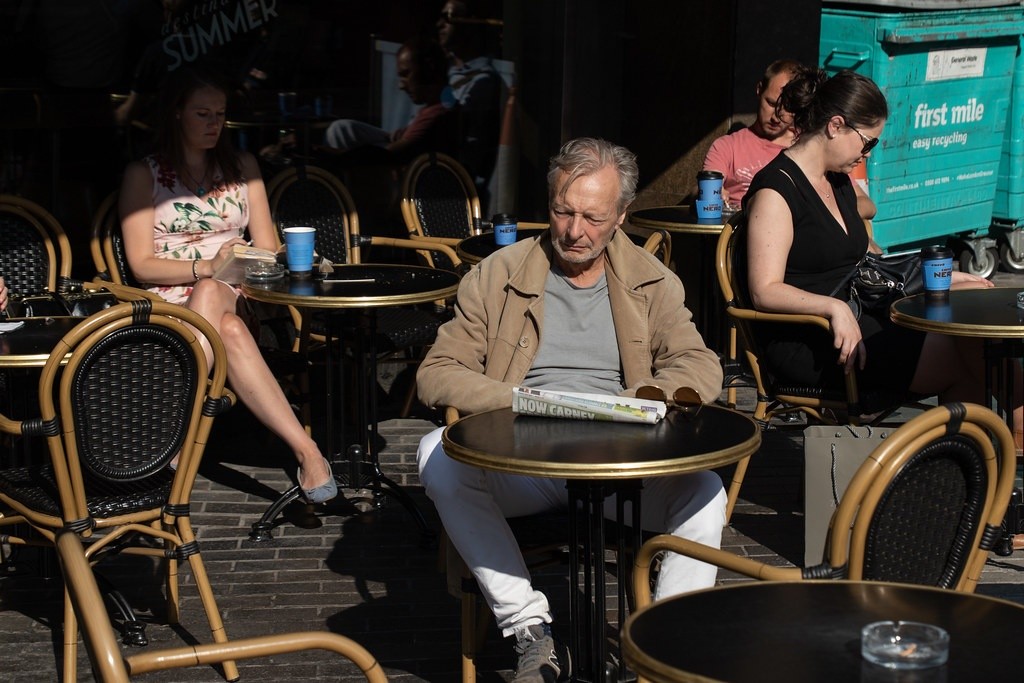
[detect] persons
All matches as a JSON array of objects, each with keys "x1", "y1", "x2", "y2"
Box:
[
  {"x1": 113, "y1": 0, "x2": 498, "y2": 187},
  {"x1": 742, "y1": 68, "x2": 1024, "y2": 455},
  {"x1": 119, "y1": 76, "x2": 338, "y2": 503},
  {"x1": 701, "y1": 58, "x2": 877, "y2": 219},
  {"x1": 417, "y1": 140, "x2": 727, "y2": 683},
  {"x1": 0, "y1": 277, "x2": 8, "y2": 311}
]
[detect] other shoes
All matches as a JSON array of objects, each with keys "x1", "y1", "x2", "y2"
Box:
[
  {"x1": 1010, "y1": 532, "x2": 1024, "y2": 550},
  {"x1": 297, "y1": 455, "x2": 337, "y2": 503},
  {"x1": 1012, "y1": 430, "x2": 1024, "y2": 456}
]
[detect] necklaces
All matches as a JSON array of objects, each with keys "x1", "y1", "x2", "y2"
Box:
[
  {"x1": 183, "y1": 160, "x2": 210, "y2": 197},
  {"x1": 787, "y1": 149, "x2": 829, "y2": 199}
]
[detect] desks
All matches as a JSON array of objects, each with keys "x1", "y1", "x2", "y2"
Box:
[
  {"x1": 628, "y1": 205, "x2": 731, "y2": 349},
  {"x1": 441, "y1": 398, "x2": 762, "y2": 683},
  {"x1": 889, "y1": 289, "x2": 1024, "y2": 556},
  {"x1": 621, "y1": 578, "x2": 1024, "y2": 683},
  {"x1": 0, "y1": 317, "x2": 147, "y2": 646},
  {"x1": 239, "y1": 263, "x2": 464, "y2": 549},
  {"x1": 456, "y1": 229, "x2": 547, "y2": 267}
]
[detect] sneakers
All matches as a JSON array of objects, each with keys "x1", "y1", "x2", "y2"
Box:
[{"x1": 513, "y1": 622, "x2": 561, "y2": 683}]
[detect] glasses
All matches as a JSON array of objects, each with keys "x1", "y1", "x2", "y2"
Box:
[{"x1": 844, "y1": 123, "x2": 879, "y2": 154}]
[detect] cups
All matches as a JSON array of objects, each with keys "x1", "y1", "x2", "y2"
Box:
[
  {"x1": 493, "y1": 212, "x2": 516, "y2": 246},
  {"x1": 283, "y1": 226, "x2": 316, "y2": 272},
  {"x1": 697, "y1": 170, "x2": 724, "y2": 201},
  {"x1": 919, "y1": 246, "x2": 953, "y2": 301}
]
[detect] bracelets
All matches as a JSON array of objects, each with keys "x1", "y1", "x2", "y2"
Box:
[{"x1": 193, "y1": 260, "x2": 198, "y2": 280}]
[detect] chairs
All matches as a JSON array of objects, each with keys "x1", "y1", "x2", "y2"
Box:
[{"x1": 1, "y1": 151, "x2": 1024, "y2": 683}]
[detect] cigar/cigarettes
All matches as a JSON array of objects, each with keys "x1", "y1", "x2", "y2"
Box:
[
  {"x1": 724, "y1": 198, "x2": 731, "y2": 210},
  {"x1": 246, "y1": 238, "x2": 254, "y2": 246}
]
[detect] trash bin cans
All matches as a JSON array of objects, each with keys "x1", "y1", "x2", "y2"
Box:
[
  {"x1": 993, "y1": 34, "x2": 1024, "y2": 274},
  {"x1": 819, "y1": 0, "x2": 1024, "y2": 280}
]
